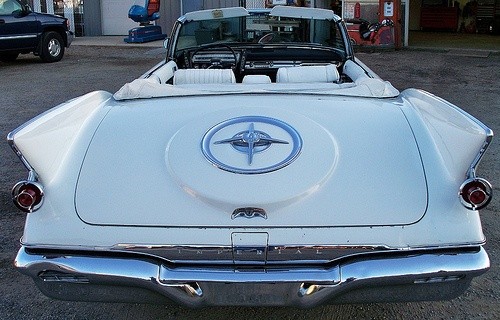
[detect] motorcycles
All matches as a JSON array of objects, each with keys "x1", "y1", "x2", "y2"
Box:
[{"x1": 336, "y1": 17, "x2": 394, "y2": 50}]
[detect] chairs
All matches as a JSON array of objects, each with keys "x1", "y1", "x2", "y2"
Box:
[
  {"x1": 173, "y1": 69, "x2": 235, "y2": 85},
  {"x1": 276, "y1": 65, "x2": 340, "y2": 84},
  {"x1": 242, "y1": 75, "x2": 271, "y2": 83}
]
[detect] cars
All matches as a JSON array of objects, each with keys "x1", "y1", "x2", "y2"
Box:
[
  {"x1": 6, "y1": 6, "x2": 495, "y2": 312},
  {"x1": 0, "y1": 0, "x2": 74, "y2": 64}
]
